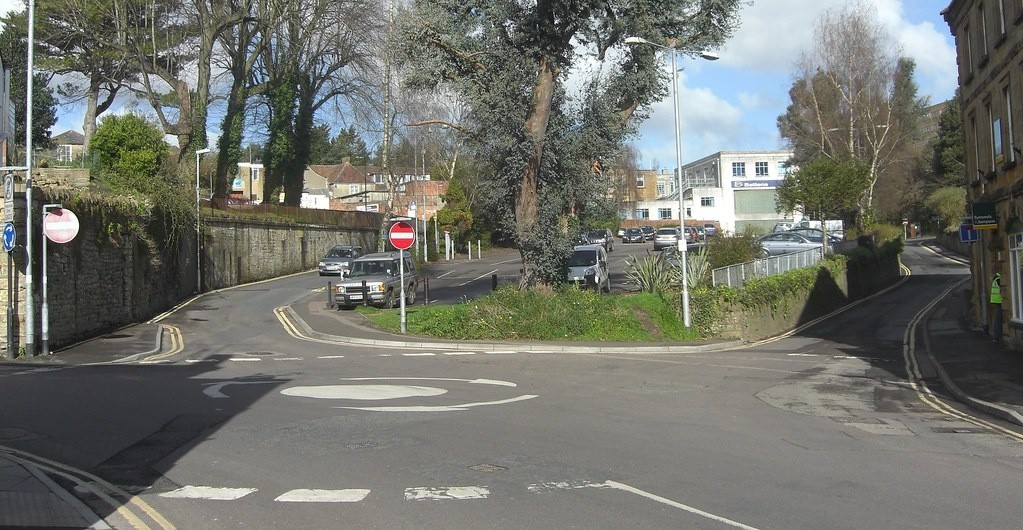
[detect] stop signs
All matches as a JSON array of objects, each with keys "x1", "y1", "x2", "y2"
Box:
[
  {"x1": 388, "y1": 221, "x2": 416, "y2": 251},
  {"x1": 42, "y1": 208, "x2": 79, "y2": 244},
  {"x1": 902, "y1": 220, "x2": 909, "y2": 226}
]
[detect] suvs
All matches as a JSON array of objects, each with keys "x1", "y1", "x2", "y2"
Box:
[
  {"x1": 317, "y1": 244, "x2": 364, "y2": 278},
  {"x1": 582, "y1": 226, "x2": 614, "y2": 254},
  {"x1": 653, "y1": 227, "x2": 681, "y2": 251},
  {"x1": 333, "y1": 250, "x2": 418, "y2": 310}
]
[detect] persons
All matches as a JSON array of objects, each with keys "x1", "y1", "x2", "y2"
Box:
[{"x1": 991, "y1": 273, "x2": 1003, "y2": 345}]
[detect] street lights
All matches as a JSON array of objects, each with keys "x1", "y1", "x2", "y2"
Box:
[
  {"x1": 829, "y1": 125, "x2": 889, "y2": 175},
  {"x1": 624, "y1": 36, "x2": 720, "y2": 328},
  {"x1": 196, "y1": 148, "x2": 211, "y2": 294}
]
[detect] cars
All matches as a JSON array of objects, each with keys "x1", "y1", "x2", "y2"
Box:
[
  {"x1": 639, "y1": 225, "x2": 657, "y2": 240},
  {"x1": 617, "y1": 227, "x2": 627, "y2": 238},
  {"x1": 752, "y1": 231, "x2": 824, "y2": 258},
  {"x1": 622, "y1": 227, "x2": 647, "y2": 244},
  {"x1": 771, "y1": 219, "x2": 847, "y2": 244},
  {"x1": 676, "y1": 225, "x2": 705, "y2": 244},
  {"x1": 702, "y1": 223, "x2": 718, "y2": 237}
]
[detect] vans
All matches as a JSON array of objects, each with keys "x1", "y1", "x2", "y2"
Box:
[{"x1": 564, "y1": 244, "x2": 610, "y2": 294}]
